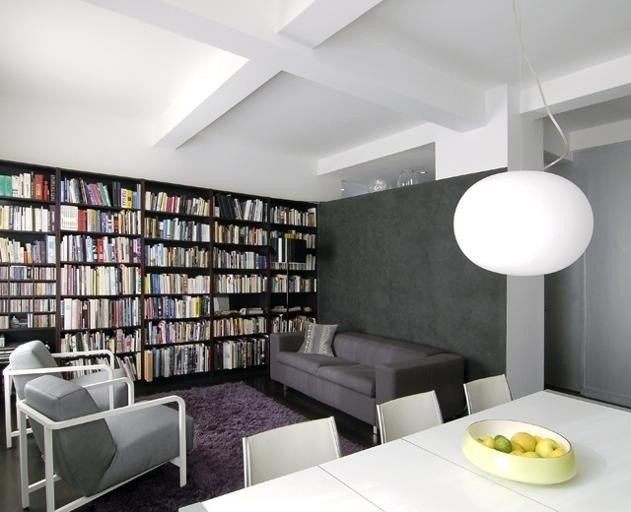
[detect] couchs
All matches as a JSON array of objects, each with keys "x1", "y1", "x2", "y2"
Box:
[{"x1": 270, "y1": 331, "x2": 465, "y2": 444}]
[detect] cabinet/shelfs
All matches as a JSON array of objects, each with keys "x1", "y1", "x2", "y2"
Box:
[{"x1": 0, "y1": 161, "x2": 320, "y2": 399}]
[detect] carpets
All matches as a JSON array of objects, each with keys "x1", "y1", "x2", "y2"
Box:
[{"x1": 92, "y1": 382, "x2": 368, "y2": 512}]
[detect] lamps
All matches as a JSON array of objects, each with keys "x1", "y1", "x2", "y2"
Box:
[{"x1": 453, "y1": 0, "x2": 593, "y2": 275}]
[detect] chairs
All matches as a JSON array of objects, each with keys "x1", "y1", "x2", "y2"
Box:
[
  {"x1": 2, "y1": 340, "x2": 128, "y2": 449},
  {"x1": 376, "y1": 390, "x2": 444, "y2": 444},
  {"x1": 464, "y1": 374, "x2": 513, "y2": 415},
  {"x1": 242, "y1": 416, "x2": 341, "y2": 487},
  {"x1": 16, "y1": 374, "x2": 194, "y2": 511}
]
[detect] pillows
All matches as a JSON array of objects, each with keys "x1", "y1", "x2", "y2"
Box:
[{"x1": 298, "y1": 321, "x2": 338, "y2": 358}]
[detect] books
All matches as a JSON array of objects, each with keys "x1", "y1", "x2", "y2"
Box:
[
  {"x1": 60, "y1": 176, "x2": 141, "y2": 381},
  {"x1": 1, "y1": 173, "x2": 56, "y2": 359},
  {"x1": 144, "y1": 190, "x2": 210, "y2": 381},
  {"x1": 212, "y1": 193, "x2": 317, "y2": 370}
]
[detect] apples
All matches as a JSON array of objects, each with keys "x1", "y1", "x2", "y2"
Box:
[
  {"x1": 508, "y1": 432, "x2": 566, "y2": 458},
  {"x1": 479, "y1": 437, "x2": 494, "y2": 448}
]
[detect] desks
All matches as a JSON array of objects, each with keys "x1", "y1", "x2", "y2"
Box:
[{"x1": 178, "y1": 389, "x2": 631, "y2": 512}]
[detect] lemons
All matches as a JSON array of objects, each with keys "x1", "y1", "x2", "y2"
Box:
[{"x1": 494, "y1": 435, "x2": 512, "y2": 453}]
